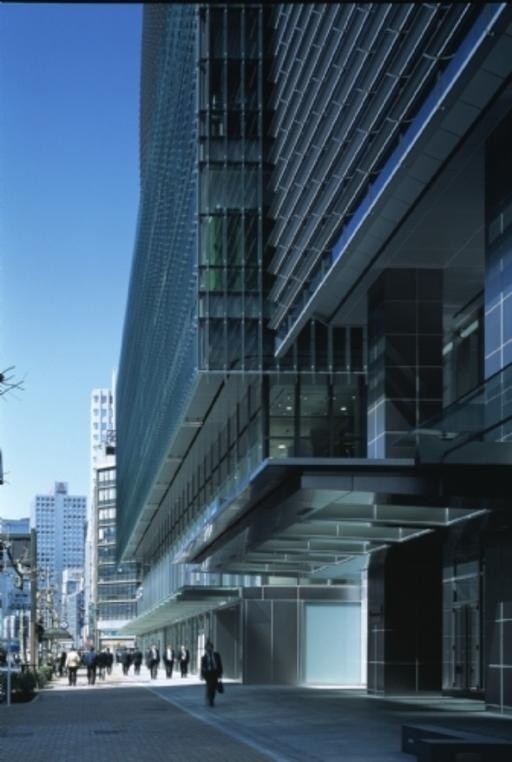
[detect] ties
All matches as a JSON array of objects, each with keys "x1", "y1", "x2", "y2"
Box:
[{"x1": 209, "y1": 655, "x2": 214, "y2": 670}]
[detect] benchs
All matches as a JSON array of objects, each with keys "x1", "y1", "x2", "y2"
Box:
[{"x1": 401, "y1": 721, "x2": 512, "y2": 762}]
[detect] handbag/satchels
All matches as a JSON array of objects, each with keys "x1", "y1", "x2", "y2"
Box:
[{"x1": 217, "y1": 682, "x2": 224, "y2": 694}]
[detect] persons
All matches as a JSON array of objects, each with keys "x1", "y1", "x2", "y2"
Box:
[
  {"x1": 57, "y1": 643, "x2": 191, "y2": 685},
  {"x1": 201, "y1": 641, "x2": 224, "y2": 708}
]
